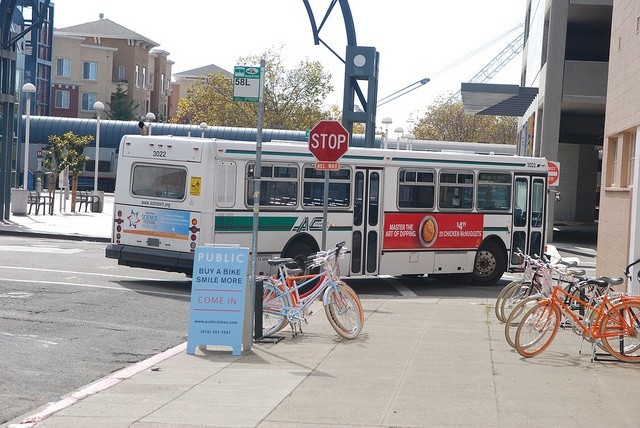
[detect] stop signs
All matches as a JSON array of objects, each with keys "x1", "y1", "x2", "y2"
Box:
[
  {"x1": 548, "y1": 160, "x2": 561, "y2": 189},
  {"x1": 308, "y1": 121, "x2": 349, "y2": 171}
]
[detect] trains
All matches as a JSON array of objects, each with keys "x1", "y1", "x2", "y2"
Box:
[{"x1": 11, "y1": 116, "x2": 603, "y2": 209}]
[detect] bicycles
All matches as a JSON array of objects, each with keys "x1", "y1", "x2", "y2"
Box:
[
  {"x1": 255, "y1": 241, "x2": 364, "y2": 340},
  {"x1": 495, "y1": 247, "x2": 640, "y2": 363}
]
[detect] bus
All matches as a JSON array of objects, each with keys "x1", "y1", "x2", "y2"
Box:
[{"x1": 106, "y1": 135, "x2": 548, "y2": 277}]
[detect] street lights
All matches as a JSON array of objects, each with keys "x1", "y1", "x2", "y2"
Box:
[
  {"x1": 22, "y1": 83, "x2": 36, "y2": 190},
  {"x1": 200, "y1": 122, "x2": 208, "y2": 138},
  {"x1": 145, "y1": 112, "x2": 156, "y2": 135},
  {"x1": 93, "y1": 101, "x2": 105, "y2": 191},
  {"x1": 394, "y1": 127, "x2": 404, "y2": 150},
  {"x1": 404, "y1": 134, "x2": 415, "y2": 150},
  {"x1": 382, "y1": 117, "x2": 392, "y2": 149},
  {"x1": 376, "y1": 77, "x2": 430, "y2": 108}
]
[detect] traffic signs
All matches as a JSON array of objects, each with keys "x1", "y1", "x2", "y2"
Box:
[{"x1": 233, "y1": 66, "x2": 261, "y2": 102}]
